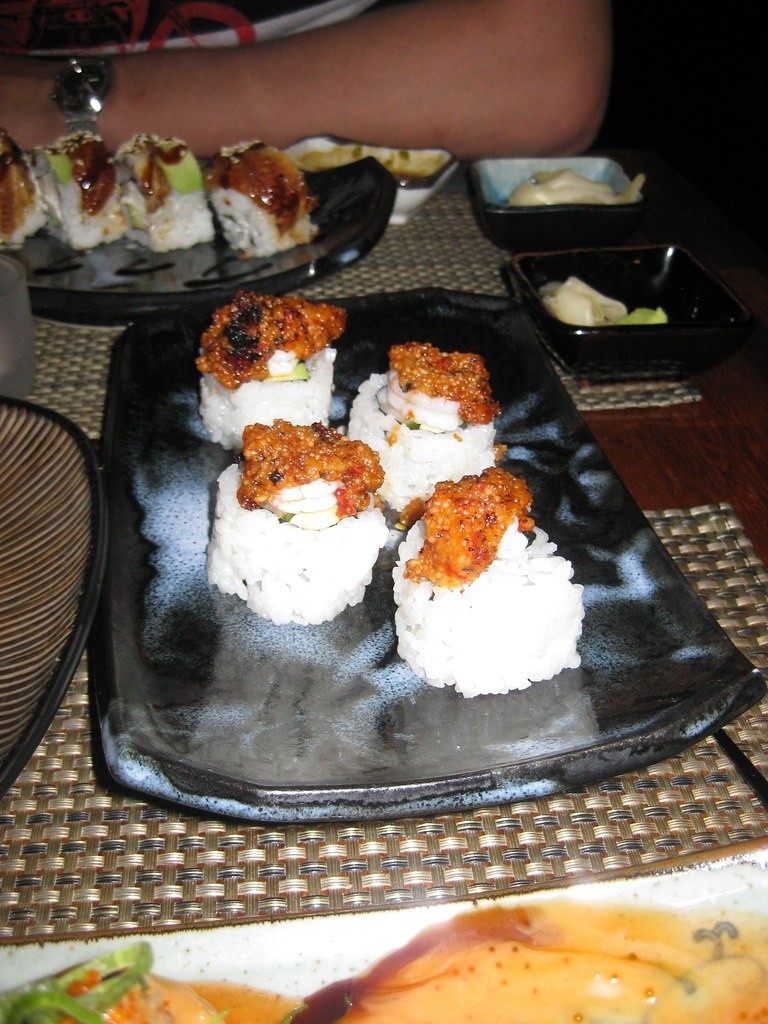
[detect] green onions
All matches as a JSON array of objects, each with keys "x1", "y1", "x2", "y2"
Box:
[{"x1": 0, "y1": 940, "x2": 153, "y2": 1024}]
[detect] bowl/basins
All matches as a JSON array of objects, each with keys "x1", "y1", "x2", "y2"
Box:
[
  {"x1": 275, "y1": 132, "x2": 458, "y2": 225},
  {"x1": 468, "y1": 154, "x2": 651, "y2": 252}
]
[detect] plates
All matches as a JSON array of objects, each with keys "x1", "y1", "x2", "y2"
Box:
[
  {"x1": 508, "y1": 243, "x2": 756, "y2": 386},
  {"x1": 1, "y1": 154, "x2": 397, "y2": 325},
  {"x1": 0, "y1": 823, "x2": 768, "y2": 1024},
  {"x1": 91, "y1": 283, "x2": 768, "y2": 820},
  {"x1": 0, "y1": 397, "x2": 103, "y2": 821}
]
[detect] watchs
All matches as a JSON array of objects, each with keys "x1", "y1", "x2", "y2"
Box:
[{"x1": 49, "y1": 54, "x2": 115, "y2": 135}]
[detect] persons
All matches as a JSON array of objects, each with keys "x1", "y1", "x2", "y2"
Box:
[{"x1": 0, "y1": 0, "x2": 613, "y2": 161}]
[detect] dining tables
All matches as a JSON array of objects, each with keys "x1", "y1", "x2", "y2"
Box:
[{"x1": 0, "y1": 149, "x2": 768, "y2": 947}]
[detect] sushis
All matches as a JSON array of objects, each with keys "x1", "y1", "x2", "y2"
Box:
[
  {"x1": 0, "y1": 127, "x2": 320, "y2": 259},
  {"x1": 194, "y1": 292, "x2": 583, "y2": 698}
]
